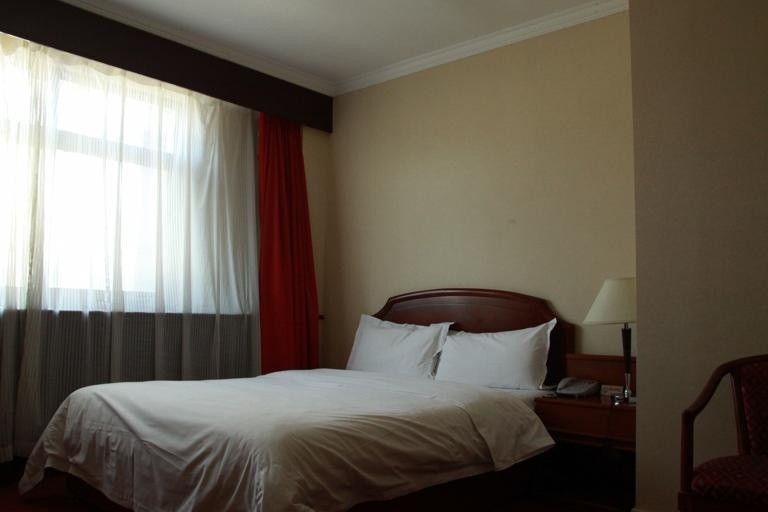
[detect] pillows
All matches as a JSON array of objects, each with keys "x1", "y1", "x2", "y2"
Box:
[
  {"x1": 435, "y1": 317, "x2": 557, "y2": 392},
  {"x1": 343, "y1": 313, "x2": 454, "y2": 380}
]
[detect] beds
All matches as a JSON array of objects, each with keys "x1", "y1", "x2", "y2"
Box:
[{"x1": 30, "y1": 288, "x2": 577, "y2": 510}]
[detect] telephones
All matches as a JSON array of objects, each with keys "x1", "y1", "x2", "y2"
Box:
[{"x1": 557, "y1": 378, "x2": 598, "y2": 396}]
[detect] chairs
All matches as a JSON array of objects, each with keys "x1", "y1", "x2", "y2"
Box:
[{"x1": 675, "y1": 349, "x2": 767, "y2": 511}]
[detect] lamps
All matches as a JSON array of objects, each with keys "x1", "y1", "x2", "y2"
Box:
[{"x1": 581, "y1": 271, "x2": 637, "y2": 404}]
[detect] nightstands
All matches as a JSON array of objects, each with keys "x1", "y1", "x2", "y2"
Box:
[{"x1": 535, "y1": 355, "x2": 637, "y2": 511}]
